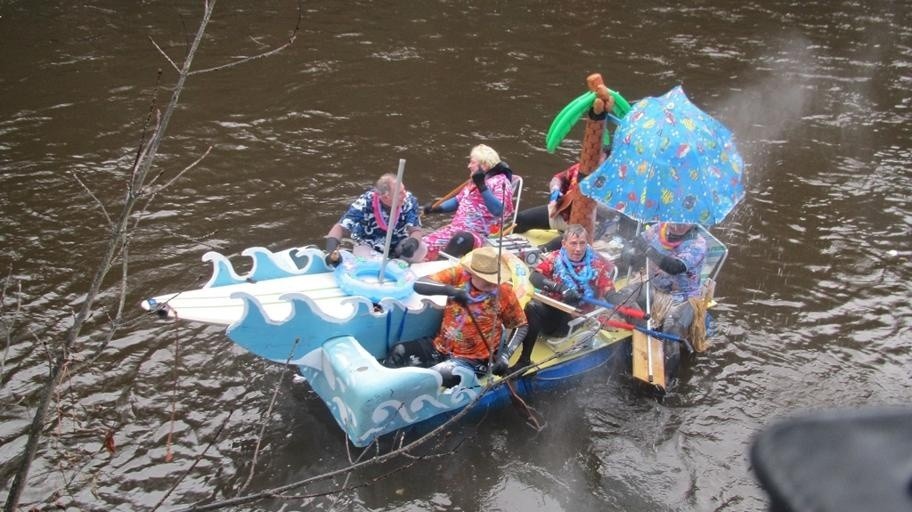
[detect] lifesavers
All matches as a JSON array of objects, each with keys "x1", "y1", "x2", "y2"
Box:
[
  {"x1": 334, "y1": 254, "x2": 417, "y2": 302},
  {"x1": 458, "y1": 246, "x2": 530, "y2": 310}
]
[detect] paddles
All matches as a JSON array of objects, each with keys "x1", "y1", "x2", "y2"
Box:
[{"x1": 632, "y1": 254, "x2": 665, "y2": 395}]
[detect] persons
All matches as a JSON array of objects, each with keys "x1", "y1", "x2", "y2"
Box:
[
  {"x1": 511, "y1": 149, "x2": 610, "y2": 233},
  {"x1": 624, "y1": 222, "x2": 708, "y2": 370},
  {"x1": 418, "y1": 143, "x2": 516, "y2": 261},
  {"x1": 511, "y1": 224, "x2": 628, "y2": 371},
  {"x1": 326, "y1": 172, "x2": 417, "y2": 267},
  {"x1": 387, "y1": 246, "x2": 532, "y2": 388}
]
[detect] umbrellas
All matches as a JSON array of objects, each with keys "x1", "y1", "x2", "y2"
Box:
[{"x1": 578, "y1": 85, "x2": 747, "y2": 238}]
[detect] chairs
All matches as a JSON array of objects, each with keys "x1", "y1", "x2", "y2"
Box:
[
  {"x1": 552, "y1": 248, "x2": 624, "y2": 358},
  {"x1": 638, "y1": 220, "x2": 728, "y2": 292},
  {"x1": 421, "y1": 173, "x2": 524, "y2": 263}
]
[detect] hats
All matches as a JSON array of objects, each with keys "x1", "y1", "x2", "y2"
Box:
[{"x1": 458, "y1": 246, "x2": 512, "y2": 287}]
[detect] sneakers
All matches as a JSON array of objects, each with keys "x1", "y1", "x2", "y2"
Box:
[{"x1": 500, "y1": 357, "x2": 534, "y2": 378}]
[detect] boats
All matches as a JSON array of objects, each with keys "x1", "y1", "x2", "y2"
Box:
[{"x1": 142, "y1": 200, "x2": 729, "y2": 449}]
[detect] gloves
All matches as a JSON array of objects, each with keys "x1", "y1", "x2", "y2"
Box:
[
  {"x1": 548, "y1": 186, "x2": 564, "y2": 203},
  {"x1": 423, "y1": 205, "x2": 444, "y2": 216},
  {"x1": 603, "y1": 285, "x2": 626, "y2": 306},
  {"x1": 401, "y1": 238, "x2": 421, "y2": 259},
  {"x1": 630, "y1": 233, "x2": 650, "y2": 254},
  {"x1": 550, "y1": 280, "x2": 582, "y2": 306},
  {"x1": 322, "y1": 235, "x2": 342, "y2": 254},
  {"x1": 471, "y1": 171, "x2": 489, "y2": 195},
  {"x1": 491, "y1": 345, "x2": 517, "y2": 376},
  {"x1": 446, "y1": 285, "x2": 471, "y2": 304}
]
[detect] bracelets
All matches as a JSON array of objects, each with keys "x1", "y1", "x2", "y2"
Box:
[
  {"x1": 557, "y1": 284, "x2": 563, "y2": 294},
  {"x1": 642, "y1": 245, "x2": 650, "y2": 253},
  {"x1": 551, "y1": 282, "x2": 558, "y2": 292},
  {"x1": 644, "y1": 246, "x2": 652, "y2": 256}
]
